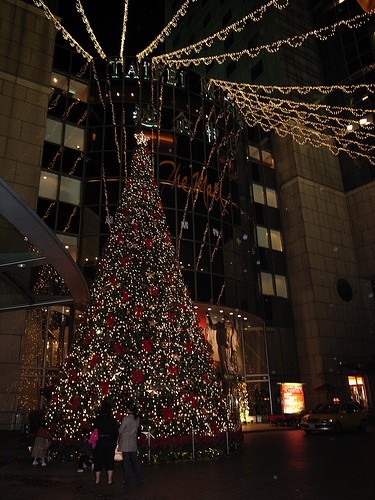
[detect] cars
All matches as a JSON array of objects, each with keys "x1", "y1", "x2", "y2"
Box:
[
  {"x1": 300, "y1": 400, "x2": 368, "y2": 435},
  {"x1": 268, "y1": 407, "x2": 311, "y2": 428}
]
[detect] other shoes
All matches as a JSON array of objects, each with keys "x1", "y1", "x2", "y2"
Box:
[
  {"x1": 32, "y1": 461, "x2": 37, "y2": 464},
  {"x1": 95, "y1": 480, "x2": 101, "y2": 485},
  {"x1": 137, "y1": 481, "x2": 145, "y2": 487},
  {"x1": 107, "y1": 480, "x2": 115, "y2": 486},
  {"x1": 41, "y1": 463, "x2": 47, "y2": 466},
  {"x1": 122, "y1": 480, "x2": 130, "y2": 487}
]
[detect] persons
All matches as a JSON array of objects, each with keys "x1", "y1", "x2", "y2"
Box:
[
  {"x1": 118, "y1": 404, "x2": 145, "y2": 487},
  {"x1": 32, "y1": 423, "x2": 51, "y2": 467},
  {"x1": 74, "y1": 408, "x2": 120, "y2": 485}
]
